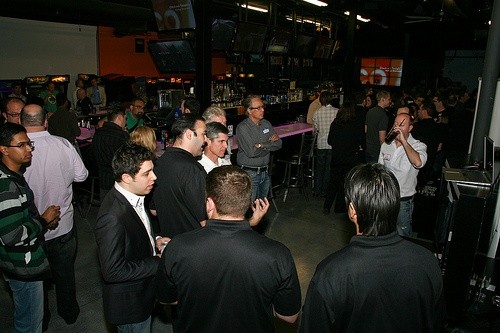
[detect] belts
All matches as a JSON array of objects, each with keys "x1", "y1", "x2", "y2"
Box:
[
  {"x1": 236, "y1": 163, "x2": 267, "y2": 172},
  {"x1": 399, "y1": 196, "x2": 413, "y2": 201}
]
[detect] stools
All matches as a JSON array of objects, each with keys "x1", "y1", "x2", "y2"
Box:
[{"x1": 273, "y1": 154, "x2": 310, "y2": 203}]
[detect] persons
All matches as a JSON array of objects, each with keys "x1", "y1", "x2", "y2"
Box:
[
  {"x1": 2, "y1": 97, "x2": 25, "y2": 125},
  {"x1": 19, "y1": 104, "x2": 89, "y2": 324},
  {"x1": 301, "y1": 163, "x2": 446, "y2": 333},
  {"x1": 94, "y1": 145, "x2": 171, "y2": 333},
  {"x1": 379, "y1": 112, "x2": 427, "y2": 237},
  {"x1": 10, "y1": 79, "x2": 107, "y2": 205},
  {"x1": 91, "y1": 95, "x2": 283, "y2": 239},
  {"x1": 306, "y1": 84, "x2": 476, "y2": 232},
  {"x1": 154, "y1": 166, "x2": 302, "y2": 333},
  {"x1": 0, "y1": 122, "x2": 61, "y2": 333}
]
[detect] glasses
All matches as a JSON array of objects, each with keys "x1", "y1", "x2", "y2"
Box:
[
  {"x1": 133, "y1": 104, "x2": 145, "y2": 111},
  {"x1": 6, "y1": 112, "x2": 20, "y2": 117},
  {"x1": 250, "y1": 105, "x2": 265, "y2": 110},
  {"x1": 3, "y1": 141, "x2": 35, "y2": 150}
]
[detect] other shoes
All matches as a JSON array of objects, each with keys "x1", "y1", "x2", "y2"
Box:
[
  {"x1": 334, "y1": 207, "x2": 348, "y2": 213},
  {"x1": 323, "y1": 208, "x2": 330, "y2": 213}
]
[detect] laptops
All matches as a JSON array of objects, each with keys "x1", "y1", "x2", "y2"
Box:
[{"x1": 443, "y1": 135, "x2": 495, "y2": 186}]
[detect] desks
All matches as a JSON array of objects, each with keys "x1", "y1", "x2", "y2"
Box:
[
  {"x1": 73, "y1": 109, "x2": 171, "y2": 158},
  {"x1": 228, "y1": 121, "x2": 313, "y2": 149}
]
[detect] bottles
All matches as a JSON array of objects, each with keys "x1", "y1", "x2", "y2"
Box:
[
  {"x1": 214, "y1": 83, "x2": 244, "y2": 108},
  {"x1": 87, "y1": 121, "x2": 90, "y2": 128},
  {"x1": 81, "y1": 120, "x2": 85, "y2": 127},
  {"x1": 262, "y1": 88, "x2": 303, "y2": 104},
  {"x1": 164, "y1": 134, "x2": 169, "y2": 150}
]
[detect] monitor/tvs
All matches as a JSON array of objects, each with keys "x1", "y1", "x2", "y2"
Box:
[{"x1": 147, "y1": 39, "x2": 198, "y2": 73}]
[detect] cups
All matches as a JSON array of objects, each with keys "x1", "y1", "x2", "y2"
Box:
[{"x1": 384, "y1": 125, "x2": 400, "y2": 145}]
